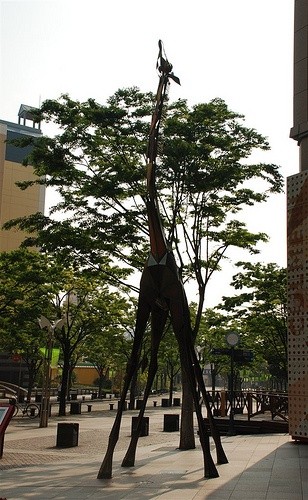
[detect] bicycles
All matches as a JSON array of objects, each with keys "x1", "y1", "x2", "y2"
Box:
[{"x1": 9, "y1": 393, "x2": 40, "y2": 419}]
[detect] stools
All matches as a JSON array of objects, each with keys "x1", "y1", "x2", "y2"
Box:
[
  {"x1": 164, "y1": 414, "x2": 180, "y2": 433},
  {"x1": 132, "y1": 416, "x2": 150, "y2": 437},
  {"x1": 161, "y1": 398, "x2": 169, "y2": 406},
  {"x1": 56, "y1": 423, "x2": 79, "y2": 448},
  {"x1": 118, "y1": 400, "x2": 128, "y2": 410},
  {"x1": 173, "y1": 398, "x2": 180, "y2": 406},
  {"x1": 136, "y1": 400, "x2": 145, "y2": 410}
]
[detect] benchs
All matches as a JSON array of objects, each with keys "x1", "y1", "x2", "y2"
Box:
[{"x1": 37, "y1": 403, "x2": 82, "y2": 416}]
